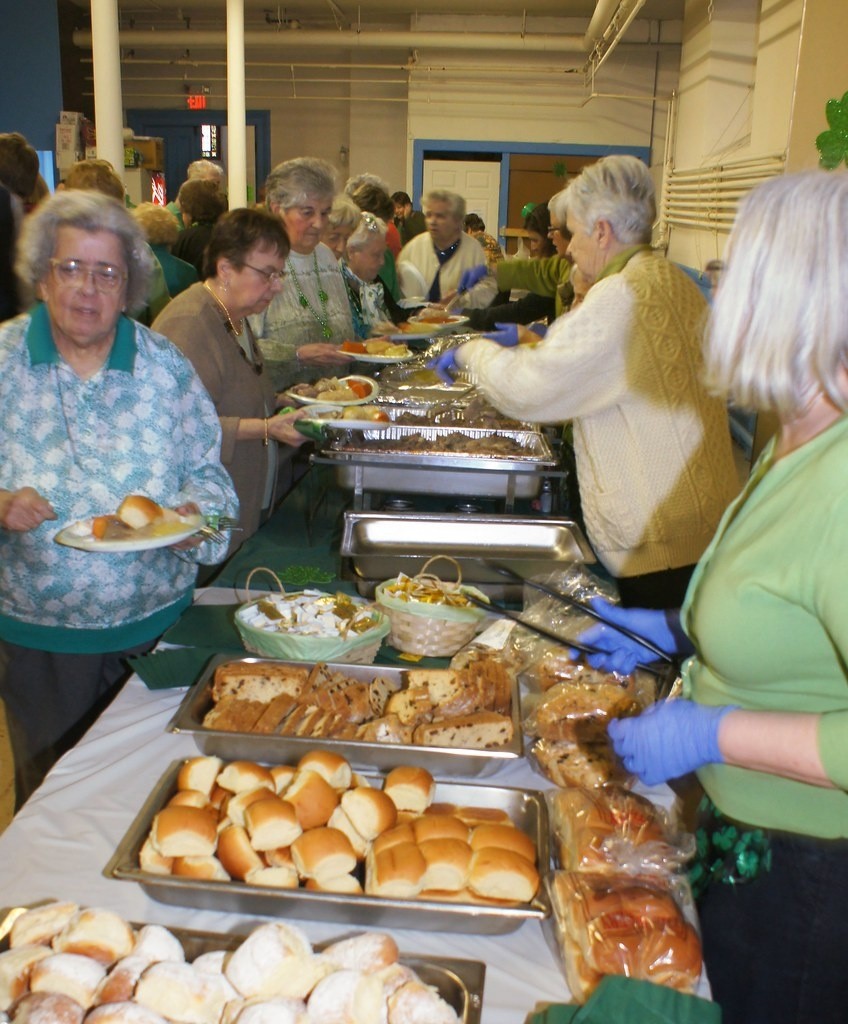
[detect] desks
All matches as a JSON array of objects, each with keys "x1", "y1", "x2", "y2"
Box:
[{"x1": 2, "y1": 463, "x2": 723, "y2": 1024}]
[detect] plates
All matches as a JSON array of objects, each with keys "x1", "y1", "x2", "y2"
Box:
[
  {"x1": 407, "y1": 315, "x2": 470, "y2": 329},
  {"x1": 367, "y1": 328, "x2": 443, "y2": 344},
  {"x1": 299, "y1": 419, "x2": 390, "y2": 429},
  {"x1": 336, "y1": 348, "x2": 414, "y2": 363},
  {"x1": 285, "y1": 375, "x2": 380, "y2": 405},
  {"x1": 54, "y1": 513, "x2": 207, "y2": 552}
]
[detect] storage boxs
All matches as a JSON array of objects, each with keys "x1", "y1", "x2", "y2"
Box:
[
  {"x1": 124, "y1": 137, "x2": 165, "y2": 172},
  {"x1": 54, "y1": 111, "x2": 98, "y2": 169}
]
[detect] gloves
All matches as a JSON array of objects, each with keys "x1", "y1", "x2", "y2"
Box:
[
  {"x1": 426, "y1": 343, "x2": 462, "y2": 386},
  {"x1": 455, "y1": 264, "x2": 489, "y2": 295},
  {"x1": 480, "y1": 322, "x2": 521, "y2": 348},
  {"x1": 606, "y1": 698, "x2": 741, "y2": 788},
  {"x1": 566, "y1": 595, "x2": 695, "y2": 674},
  {"x1": 529, "y1": 322, "x2": 549, "y2": 338}
]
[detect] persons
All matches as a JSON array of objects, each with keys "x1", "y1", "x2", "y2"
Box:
[
  {"x1": 396, "y1": 190, "x2": 488, "y2": 303},
  {"x1": 0, "y1": 133, "x2": 229, "y2": 343},
  {"x1": 426, "y1": 156, "x2": 742, "y2": 606},
  {"x1": 148, "y1": 207, "x2": 316, "y2": 587},
  {"x1": 568, "y1": 171, "x2": 848, "y2": 1024},
  {"x1": 0, "y1": 190, "x2": 239, "y2": 817},
  {"x1": 251, "y1": 152, "x2": 427, "y2": 393}
]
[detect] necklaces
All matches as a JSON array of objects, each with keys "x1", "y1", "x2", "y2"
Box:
[
  {"x1": 205, "y1": 281, "x2": 244, "y2": 335},
  {"x1": 285, "y1": 250, "x2": 334, "y2": 343}
]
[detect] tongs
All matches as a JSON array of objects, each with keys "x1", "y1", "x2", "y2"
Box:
[{"x1": 465, "y1": 559, "x2": 679, "y2": 677}]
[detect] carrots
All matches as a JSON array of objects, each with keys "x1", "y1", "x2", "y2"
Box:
[
  {"x1": 372, "y1": 410, "x2": 389, "y2": 423},
  {"x1": 346, "y1": 379, "x2": 373, "y2": 399},
  {"x1": 340, "y1": 341, "x2": 369, "y2": 353}
]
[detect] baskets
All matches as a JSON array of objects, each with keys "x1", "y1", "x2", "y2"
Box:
[
  {"x1": 234, "y1": 567, "x2": 391, "y2": 666},
  {"x1": 374, "y1": 554, "x2": 489, "y2": 658}
]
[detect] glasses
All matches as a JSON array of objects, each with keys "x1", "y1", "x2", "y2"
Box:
[
  {"x1": 547, "y1": 227, "x2": 565, "y2": 234},
  {"x1": 46, "y1": 260, "x2": 132, "y2": 295},
  {"x1": 244, "y1": 263, "x2": 292, "y2": 287}
]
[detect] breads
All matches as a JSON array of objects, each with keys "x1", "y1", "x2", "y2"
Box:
[
  {"x1": 116, "y1": 495, "x2": 163, "y2": 529},
  {"x1": 0, "y1": 902, "x2": 462, "y2": 1024},
  {"x1": 525, "y1": 650, "x2": 705, "y2": 995},
  {"x1": 137, "y1": 750, "x2": 538, "y2": 909},
  {"x1": 203, "y1": 641, "x2": 513, "y2": 749}
]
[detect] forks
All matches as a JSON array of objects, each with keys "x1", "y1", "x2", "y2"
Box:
[
  {"x1": 205, "y1": 516, "x2": 240, "y2": 532},
  {"x1": 198, "y1": 524, "x2": 229, "y2": 545}
]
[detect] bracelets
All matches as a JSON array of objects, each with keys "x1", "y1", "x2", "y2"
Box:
[{"x1": 263, "y1": 418, "x2": 268, "y2": 449}]
